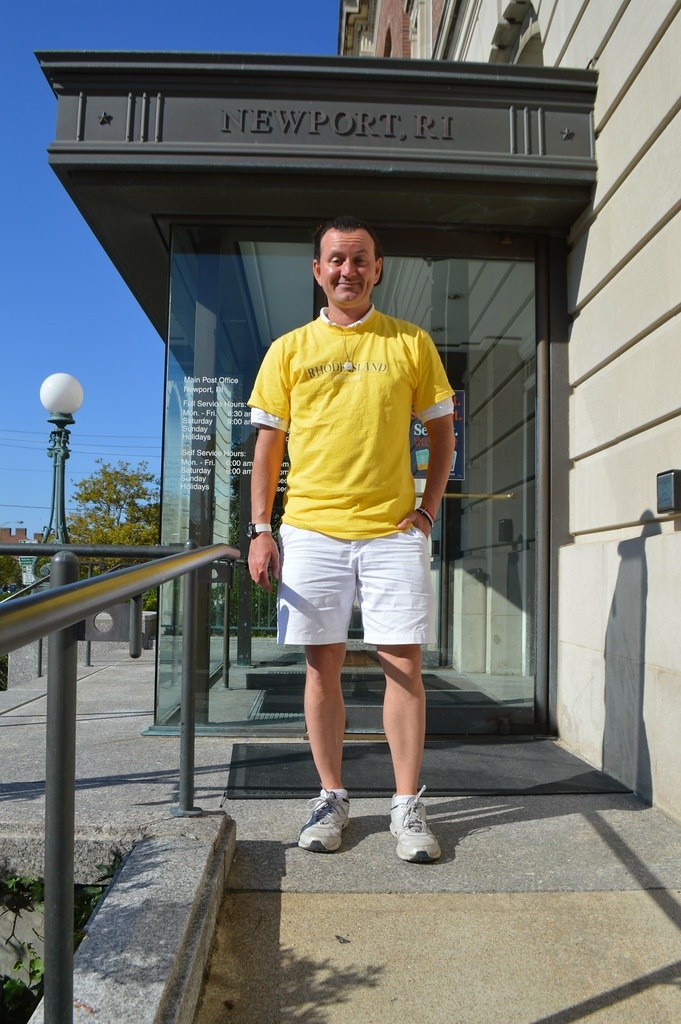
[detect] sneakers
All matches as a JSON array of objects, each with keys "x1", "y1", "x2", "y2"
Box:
[
  {"x1": 298, "y1": 783, "x2": 350, "y2": 853},
  {"x1": 389, "y1": 785, "x2": 442, "y2": 862}
]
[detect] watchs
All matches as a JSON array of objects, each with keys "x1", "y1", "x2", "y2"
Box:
[{"x1": 246, "y1": 522, "x2": 271, "y2": 538}]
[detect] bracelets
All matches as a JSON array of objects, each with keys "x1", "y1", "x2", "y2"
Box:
[{"x1": 417, "y1": 507, "x2": 434, "y2": 526}]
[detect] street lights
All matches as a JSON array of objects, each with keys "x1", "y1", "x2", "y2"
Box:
[{"x1": 33, "y1": 373, "x2": 83, "y2": 582}]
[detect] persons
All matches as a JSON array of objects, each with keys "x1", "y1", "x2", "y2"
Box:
[{"x1": 248, "y1": 215, "x2": 456, "y2": 860}]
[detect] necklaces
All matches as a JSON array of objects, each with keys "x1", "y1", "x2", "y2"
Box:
[{"x1": 340, "y1": 326, "x2": 355, "y2": 370}]
